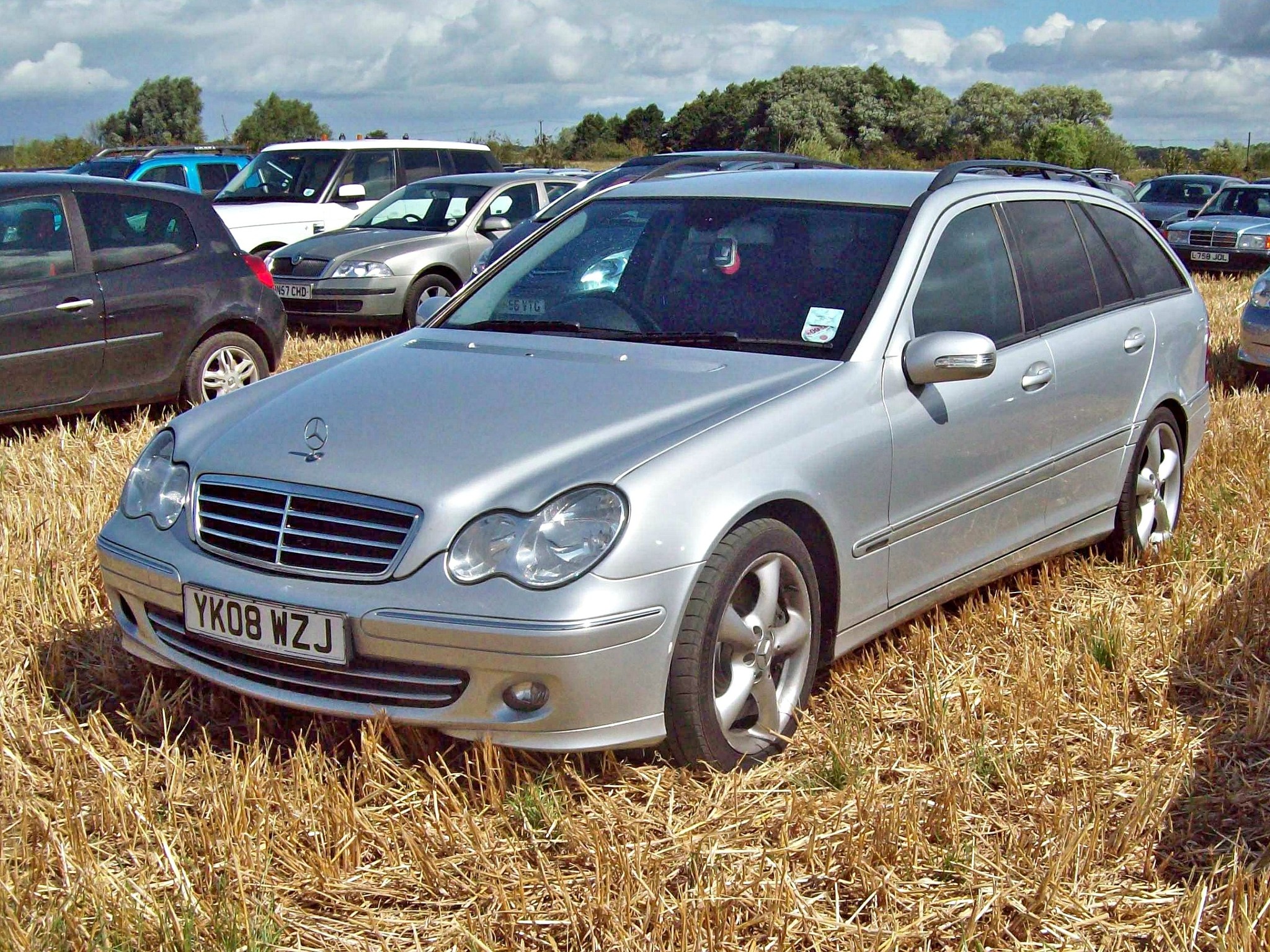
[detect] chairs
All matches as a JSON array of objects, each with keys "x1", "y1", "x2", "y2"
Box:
[
  {"x1": 18, "y1": 208, "x2": 55, "y2": 249},
  {"x1": 465, "y1": 196, "x2": 490, "y2": 217},
  {"x1": 1187, "y1": 187, "x2": 1204, "y2": 197},
  {"x1": 1239, "y1": 194, "x2": 1259, "y2": 214}
]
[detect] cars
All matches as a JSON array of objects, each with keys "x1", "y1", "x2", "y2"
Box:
[
  {"x1": 465, "y1": 149, "x2": 864, "y2": 323},
  {"x1": 503, "y1": 162, "x2": 609, "y2": 201},
  {"x1": 1133, "y1": 175, "x2": 1270, "y2": 240},
  {"x1": 259, "y1": 172, "x2": 592, "y2": 333},
  {"x1": 0, "y1": 171, "x2": 288, "y2": 422},
  {"x1": 1164, "y1": 184, "x2": 1270, "y2": 268},
  {"x1": 1236, "y1": 266, "x2": 1270, "y2": 371}
]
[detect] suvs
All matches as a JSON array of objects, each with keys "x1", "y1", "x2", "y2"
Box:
[
  {"x1": 0, "y1": 145, "x2": 267, "y2": 247},
  {"x1": 97, "y1": 157, "x2": 1209, "y2": 782},
  {"x1": 1021, "y1": 166, "x2": 1146, "y2": 233}
]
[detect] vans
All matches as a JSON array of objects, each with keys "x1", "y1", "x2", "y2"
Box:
[{"x1": 212, "y1": 138, "x2": 514, "y2": 265}]
